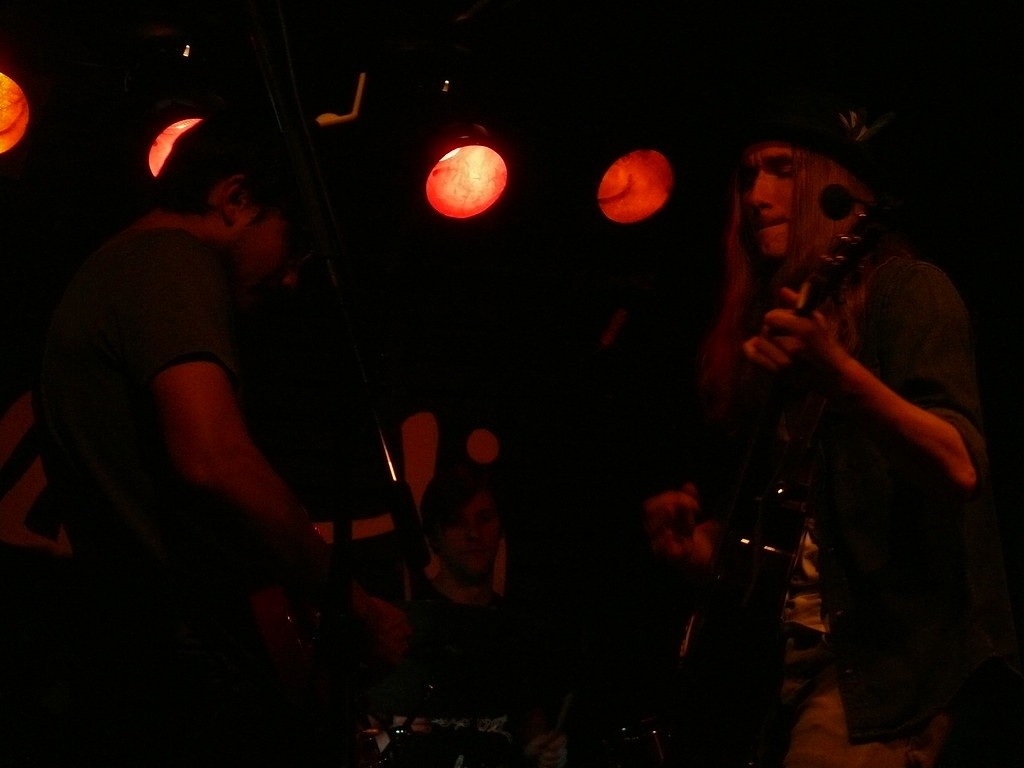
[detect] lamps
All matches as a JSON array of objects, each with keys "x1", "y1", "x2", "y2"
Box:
[{"x1": 417, "y1": 123, "x2": 509, "y2": 223}]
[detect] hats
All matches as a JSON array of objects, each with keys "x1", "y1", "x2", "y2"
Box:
[{"x1": 758, "y1": 88, "x2": 887, "y2": 196}]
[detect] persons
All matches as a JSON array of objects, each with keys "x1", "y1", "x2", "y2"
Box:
[
  {"x1": 641, "y1": 95, "x2": 1017, "y2": 768},
  {"x1": 356, "y1": 465, "x2": 567, "y2": 768},
  {"x1": 41, "y1": 93, "x2": 414, "y2": 768}
]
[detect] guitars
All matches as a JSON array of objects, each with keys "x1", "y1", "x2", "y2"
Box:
[{"x1": 684, "y1": 194, "x2": 902, "y2": 684}]
